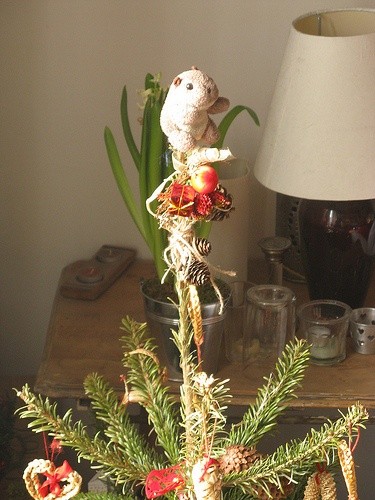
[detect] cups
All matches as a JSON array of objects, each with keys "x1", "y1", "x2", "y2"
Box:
[
  {"x1": 296, "y1": 299, "x2": 352, "y2": 366},
  {"x1": 224, "y1": 281, "x2": 256, "y2": 365},
  {"x1": 242, "y1": 284, "x2": 297, "y2": 382}
]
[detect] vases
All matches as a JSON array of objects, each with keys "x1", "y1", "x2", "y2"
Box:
[{"x1": 139, "y1": 274, "x2": 232, "y2": 383}]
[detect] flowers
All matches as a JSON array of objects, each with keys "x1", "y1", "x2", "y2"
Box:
[{"x1": 105, "y1": 72, "x2": 261, "y2": 294}]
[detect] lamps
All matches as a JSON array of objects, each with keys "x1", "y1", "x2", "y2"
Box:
[{"x1": 252, "y1": 7, "x2": 375, "y2": 319}]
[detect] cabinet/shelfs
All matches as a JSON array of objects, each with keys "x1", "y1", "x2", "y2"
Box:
[{"x1": 33, "y1": 257, "x2": 375, "y2": 500}]
